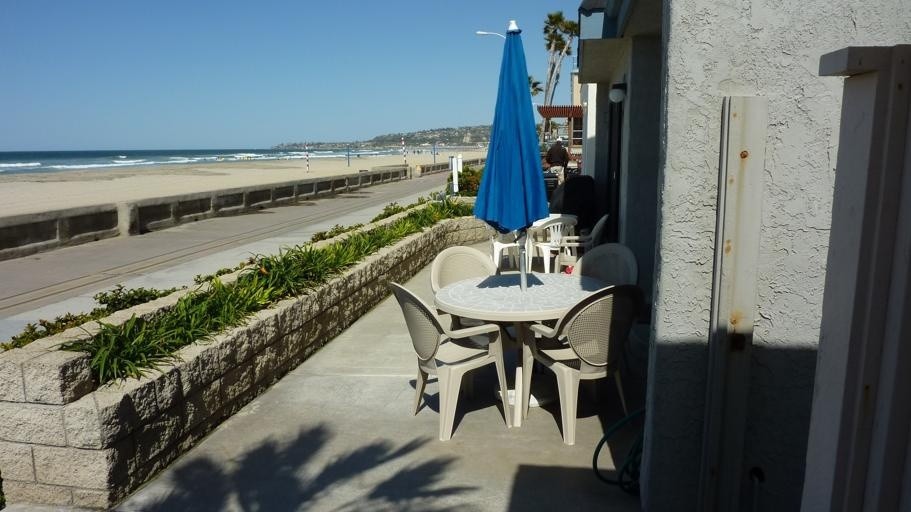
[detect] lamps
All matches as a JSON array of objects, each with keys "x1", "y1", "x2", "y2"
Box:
[{"x1": 608, "y1": 82, "x2": 627, "y2": 103}]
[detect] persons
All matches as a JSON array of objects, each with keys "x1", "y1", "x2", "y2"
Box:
[
  {"x1": 544, "y1": 137, "x2": 568, "y2": 189},
  {"x1": 407, "y1": 150, "x2": 440, "y2": 155}
]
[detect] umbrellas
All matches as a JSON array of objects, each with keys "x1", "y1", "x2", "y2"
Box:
[{"x1": 470, "y1": 18, "x2": 550, "y2": 294}]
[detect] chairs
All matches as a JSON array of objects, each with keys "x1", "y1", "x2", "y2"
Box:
[
  {"x1": 388, "y1": 281, "x2": 512, "y2": 441},
  {"x1": 571, "y1": 243, "x2": 639, "y2": 287},
  {"x1": 522, "y1": 283, "x2": 643, "y2": 447},
  {"x1": 488, "y1": 202, "x2": 609, "y2": 274},
  {"x1": 431, "y1": 246, "x2": 500, "y2": 329}
]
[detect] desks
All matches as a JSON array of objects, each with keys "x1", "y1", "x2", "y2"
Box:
[{"x1": 434, "y1": 272, "x2": 609, "y2": 427}]
